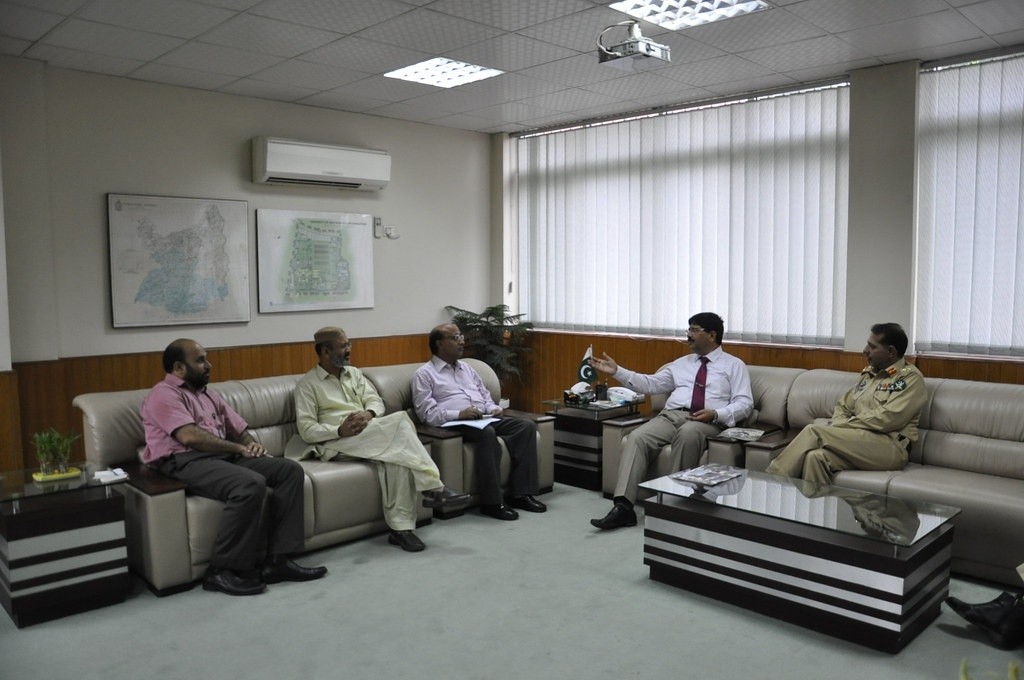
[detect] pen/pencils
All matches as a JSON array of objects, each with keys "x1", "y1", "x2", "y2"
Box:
[
  {"x1": 482, "y1": 415, "x2": 493, "y2": 418},
  {"x1": 107, "y1": 467, "x2": 119, "y2": 476},
  {"x1": 352, "y1": 387, "x2": 357, "y2": 396}
]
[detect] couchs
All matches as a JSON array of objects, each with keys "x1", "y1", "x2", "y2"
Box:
[
  {"x1": 602, "y1": 364, "x2": 1024, "y2": 588},
  {"x1": 73, "y1": 359, "x2": 556, "y2": 597}
]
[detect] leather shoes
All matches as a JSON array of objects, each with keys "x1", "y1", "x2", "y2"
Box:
[
  {"x1": 942, "y1": 588, "x2": 1024, "y2": 651},
  {"x1": 203, "y1": 570, "x2": 265, "y2": 596},
  {"x1": 421, "y1": 486, "x2": 472, "y2": 507},
  {"x1": 389, "y1": 529, "x2": 425, "y2": 553},
  {"x1": 590, "y1": 504, "x2": 637, "y2": 529},
  {"x1": 263, "y1": 558, "x2": 329, "y2": 585},
  {"x1": 475, "y1": 503, "x2": 519, "y2": 521},
  {"x1": 503, "y1": 492, "x2": 547, "y2": 512}
]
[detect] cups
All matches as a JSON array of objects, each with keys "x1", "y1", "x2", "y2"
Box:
[{"x1": 596, "y1": 385, "x2": 607, "y2": 399}]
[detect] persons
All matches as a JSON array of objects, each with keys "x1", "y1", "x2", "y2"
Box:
[
  {"x1": 284, "y1": 327, "x2": 473, "y2": 552},
  {"x1": 587, "y1": 313, "x2": 754, "y2": 529},
  {"x1": 412, "y1": 323, "x2": 546, "y2": 520},
  {"x1": 138, "y1": 339, "x2": 327, "y2": 596},
  {"x1": 764, "y1": 323, "x2": 927, "y2": 485},
  {"x1": 945, "y1": 562, "x2": 1024, "y2": 648}
]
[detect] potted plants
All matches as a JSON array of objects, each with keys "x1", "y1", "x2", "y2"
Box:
[{"x1": 33, "y1": 430, "x2": 79, "y2": 474}]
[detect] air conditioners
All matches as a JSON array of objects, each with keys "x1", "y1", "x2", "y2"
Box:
[{"x1": 251, "y1": 136, "x2": 391, "y2": 192}]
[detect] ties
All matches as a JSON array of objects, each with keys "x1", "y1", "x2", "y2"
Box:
[{"x1": 690, "y1": 356, "x2": 711, "y2": 418}]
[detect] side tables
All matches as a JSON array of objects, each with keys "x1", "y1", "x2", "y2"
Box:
[
  {"x1": 542, "y1": 393, "x2": 644, "y2": 492},
  {"x1": 1, "y1": 467, "x2": 128, "y2": 630}
]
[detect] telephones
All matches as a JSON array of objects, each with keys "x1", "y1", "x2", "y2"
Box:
[{"x1": 607, "y1": 386, "x2": 645, "y2": 404}]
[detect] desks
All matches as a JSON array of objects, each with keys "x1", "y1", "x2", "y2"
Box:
[{"x1": 637, "y1": 463, "x2": 961, "y2": 655}]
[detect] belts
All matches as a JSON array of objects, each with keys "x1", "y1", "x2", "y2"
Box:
[{"x1": 672, "y1": 407, "x2": 690, "y2": 413}]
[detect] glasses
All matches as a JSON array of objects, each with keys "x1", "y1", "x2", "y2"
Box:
[
  {"x1": 441, "y1": 335, "x2": 464, "y2": 341},
  {"x1": 332, "y1": 339, "x2": 352, "y2": 349}
]
